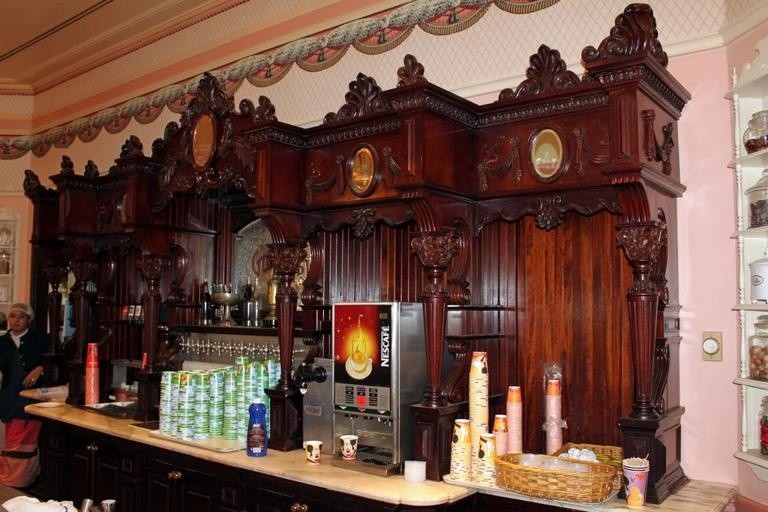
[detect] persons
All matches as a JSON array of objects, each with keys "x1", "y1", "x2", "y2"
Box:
[{"x1": 0, "y1": 302, "x2": 52, "y2": 488}]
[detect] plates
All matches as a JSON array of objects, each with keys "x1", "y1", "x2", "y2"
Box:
[{"x1": 345, "y1": 356, "x2": 373, "y2": 380}]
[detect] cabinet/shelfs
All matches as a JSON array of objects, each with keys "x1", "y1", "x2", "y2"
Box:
[
  {"x1": 0, "y1": 208, "x2": 21, "y2": 336},
  {"x1": 724, "y1": 36, "x2": 768, "y2": 512}
]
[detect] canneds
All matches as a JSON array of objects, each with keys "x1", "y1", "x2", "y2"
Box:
[{"x1": 159, "y1": 357, "x2": 281, "y2": 440}]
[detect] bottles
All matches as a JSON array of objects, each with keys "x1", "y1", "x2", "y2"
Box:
[
  {"x1": 745, "y1": 168, "x2": 767, "y2": 229},
  {"x1": 748, "y1": 248, "x2": 767, "y2": 306},
  {"x1": 743, "y1": 109, "x2": 768, "y2": 154},
  {"x1": 200, "y1": 275, "x2": 264, "y2": 326},
  {"x1": 747, "y1": 315, "x2": 768, "y2": 382},
  {"x1": 246, "y1": 398, "x2": 267, "y2": 457}
]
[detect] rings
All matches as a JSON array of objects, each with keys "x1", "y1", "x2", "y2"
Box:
[{"x1": 31, "y1": 378, "x2": 35, "y2": 382}]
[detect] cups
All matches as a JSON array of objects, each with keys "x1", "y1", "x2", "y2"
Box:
[
  {"x1": 157, "y1": 355, "x2": 282, "y2": 442},
  {"x1": 303, "y1": 439, "x2": 323, "y2": 465},
  {"x1": 449, "y1": 351, "x2": 523, "y2": 487},
  {"x1": 339, "y1": 434, "x2": 358, "y2": 461},
  {"x1": 622, "y1": 456, "x2": 650, "y2": 509},
  {"x1": 84, "y1": 343, "x2": 100, "y2": 407},
  {"x1": 545, "y1": 379, "x2": 563, "y2": 456}
]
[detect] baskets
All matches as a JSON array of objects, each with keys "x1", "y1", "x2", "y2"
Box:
[
  {"x1": 553, "y1": 441, "x2": 626, "y2": 491},
  {"x1": 494, "y1": 453, "x2": 617, "y2": 502}
]
